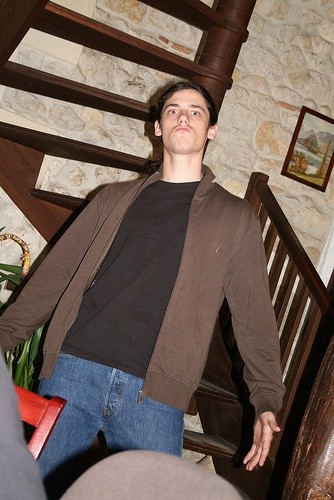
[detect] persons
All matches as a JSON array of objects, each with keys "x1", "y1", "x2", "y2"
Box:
[{"x1": 0, "y1": 80, "x2": 287, "y2": 500}]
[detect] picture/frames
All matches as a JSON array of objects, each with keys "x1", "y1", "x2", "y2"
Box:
[{"x1": 280, "y1": 105, "x2": 334, "y2": 192}]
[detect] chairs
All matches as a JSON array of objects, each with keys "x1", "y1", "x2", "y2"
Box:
[{"x1": 13, "y1": 383, "x2": 69, "y2": 461}]
